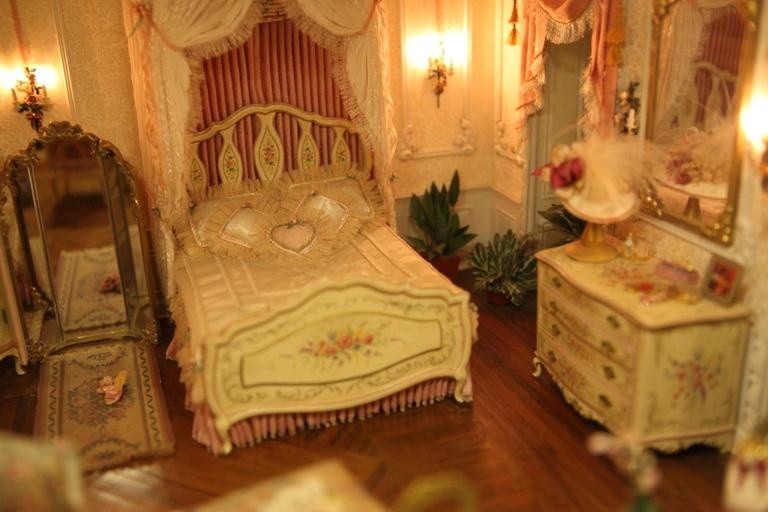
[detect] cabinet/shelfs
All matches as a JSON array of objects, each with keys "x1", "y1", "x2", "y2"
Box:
[{"x1": 532, "y1": 241, "x2": 752, "y2": 478}]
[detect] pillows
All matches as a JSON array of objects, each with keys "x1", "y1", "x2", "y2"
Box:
[{"x1": 175, "y1": 157, "x2": 392, "y2": 272}]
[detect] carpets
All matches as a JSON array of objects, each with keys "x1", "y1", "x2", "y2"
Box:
[
  {"x1": 31, "y1": 343, "x2": 175, "y2": 474},
  {"x1": 56, "y1": 245, "x2": 127, "y2": 333}
]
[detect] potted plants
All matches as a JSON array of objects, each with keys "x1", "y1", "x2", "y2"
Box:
[{"x1": 406, "y1": 167, "x2": 538, "y2": 309}]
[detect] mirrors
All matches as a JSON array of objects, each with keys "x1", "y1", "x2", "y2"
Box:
[
  {"x1": 637, "y1": 1, "x2": 764, "y2": 246},
  {"x1": 0, "y1": 120, "x2": 166, "y2": 366}
]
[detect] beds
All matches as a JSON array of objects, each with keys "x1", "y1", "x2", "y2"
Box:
[{"x1": 159, "y1": 103, "x2": 479, "y2": 457}]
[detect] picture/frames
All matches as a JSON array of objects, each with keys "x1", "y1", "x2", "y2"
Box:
[{"x1": 701, "y1": 253, "x2": 744, "y2": 307}]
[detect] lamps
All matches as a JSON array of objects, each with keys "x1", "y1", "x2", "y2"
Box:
[{"x1": 1, "y1": 65, "x2": 60, "y2": 135}]
